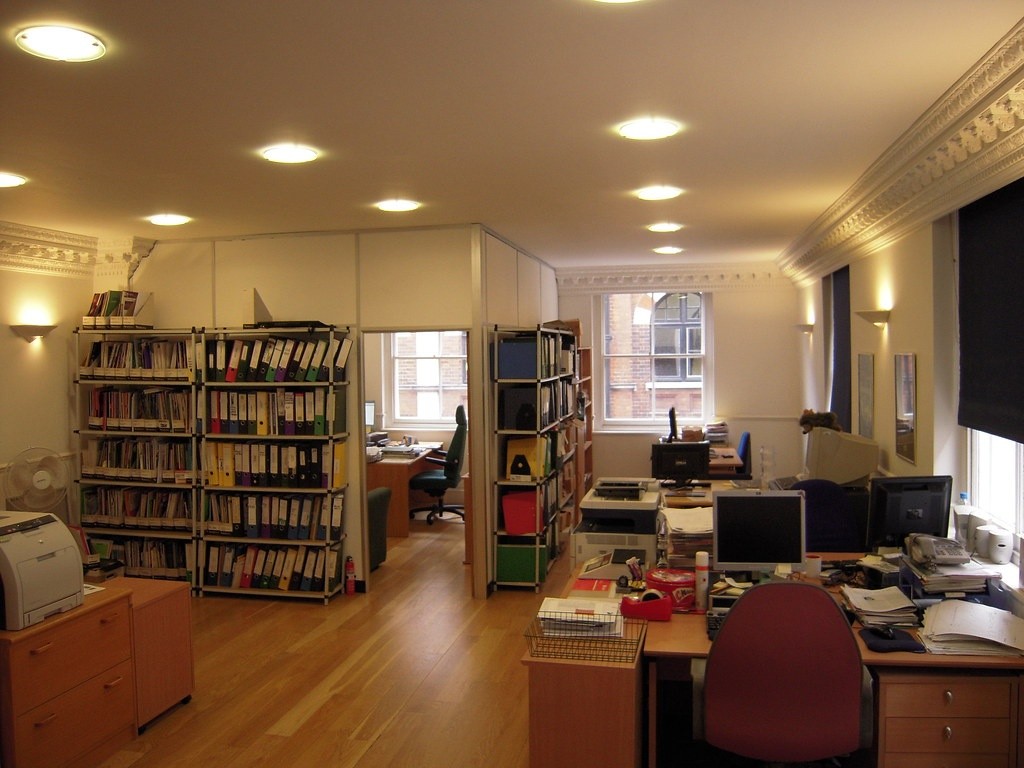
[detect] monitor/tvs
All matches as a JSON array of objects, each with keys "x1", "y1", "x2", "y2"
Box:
[
  {"x1": 666, "y1": 407, "x2": 677, "y2": 444},
  {"x1": 865, "y1": 475, "x2": 952, "y2": 555},
  {"x1": 712, "y1": 489, "x2": 806, "y2": 588},
  {"x1": 651, "y1": 441, "x2": 710, "y2": 489},
  {"x1": 364, "y1": 400, "x2": 376, "y2": 426},
  {"x1": 796, "y1": 427, "x2": 881, "y2": 483}
]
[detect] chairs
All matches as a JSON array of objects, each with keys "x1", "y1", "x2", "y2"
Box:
[
  {"x1": 68, "y1": 526, "x2": 125, "y2": 577},
  {"x1": 709, "y1": 432, "x2": 754, "y2": 480},
  {"x1": 408, "y1": 405, "x2": 467, "y2": 526},
  {"x1": 689, "y1": 580, "x2": 875, "y2": 768}
]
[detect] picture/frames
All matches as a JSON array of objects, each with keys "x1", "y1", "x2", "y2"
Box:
[
  {"x1": 893, "y1": 352, "x2": 917, "y2": 467},
  {"x1": 857, "y1": 352, "x2": 874, "y2": 441}
]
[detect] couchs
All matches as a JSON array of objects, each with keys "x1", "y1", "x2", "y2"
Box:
[{"x1": 367, "y1": 487, "x2": 391, "y2": 573}]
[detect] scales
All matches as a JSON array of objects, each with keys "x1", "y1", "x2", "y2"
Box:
[{"x1": 578, "y1": 548, "x2": 647, "y2": 580}]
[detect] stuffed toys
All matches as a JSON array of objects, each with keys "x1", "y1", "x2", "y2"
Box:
[{"x1": 800, "y1": 409, "x2": 841, "y2": 434}]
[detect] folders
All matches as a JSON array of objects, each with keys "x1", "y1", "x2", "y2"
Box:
[
  {"x1": 205, "y1": 335, "x2": 353, "y2": 383},
  {"x1": 218, "y1": 543, "x2": 309, "y2": 591},
  {"x1": 206, "y1": 441, "x2": 348, "y2": 487},
  {"x1": 299, "y1": 548, "x2": 318, "y2": 591},
  {"x1": 211, "y1": 386, "x2": 346, "y2": 435},
  {"x1": 329, "y1": 542, "x2": 342, "y2": 592},
  {"x1": 205, "y1": 491, "x2": 344, "y2": 540},
  {"x1": 312, "y1": 548, "x2": 325, "y2": 591},
  {"x1": 205, "y1": 545, "x2": 219, "y2": 586}
]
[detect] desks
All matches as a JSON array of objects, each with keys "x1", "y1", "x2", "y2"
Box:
[
  {"x1": 659, "y1": 476, "x2": 776, "y2": 508},
  {"x1": 368, "y1": 441, "x2": 443, "y2": 538},
  {"x1": 643, "y1": 551, "x2": 1024, "y2": 768},
  {"x1": 709, "y1": 447, "x2": 744, "y2": 476}
]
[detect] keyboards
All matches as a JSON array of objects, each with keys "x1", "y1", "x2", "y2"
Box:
[
  {"x1": 661, "y1": 481, "x2": 711, "y2": 488},
  {"x1": 769, "y1": 477, "x2": 800, "y2": 491}
]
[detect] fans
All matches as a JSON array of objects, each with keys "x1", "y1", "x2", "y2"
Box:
[{"x1": 3, "y1": 444, "x2": 70, "y2": 514}]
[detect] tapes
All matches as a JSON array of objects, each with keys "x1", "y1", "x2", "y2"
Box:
[{"x1": 640, "y1": 589, "x2": 663, "y2": 602}]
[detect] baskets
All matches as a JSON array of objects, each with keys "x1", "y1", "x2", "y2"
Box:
[{"x1": 522, "y1": 610, "x2": 648, "y2": 665}]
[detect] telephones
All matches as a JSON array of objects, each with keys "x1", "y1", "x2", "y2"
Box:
[{"x1": 905, "y1": 533, "x2": 970, "y2": 567}]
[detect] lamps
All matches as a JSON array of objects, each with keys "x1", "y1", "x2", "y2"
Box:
[
  {"x1": 853, "y1": 310, "x2": 890, "y2": 324},
  {"x1": 9, "y1": 323, "x2": 59, "y2": 345},
  {"x1": 798, "y1": 325, "x2": 814, "y2": 334}
]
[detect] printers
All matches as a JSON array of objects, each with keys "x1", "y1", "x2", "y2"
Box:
[
  {"x1": 571, "y1": 476, "x2": 662, "y2": 573},
  {"x1": 0, "y1": 510, "x2": 84, "y2": 631}
]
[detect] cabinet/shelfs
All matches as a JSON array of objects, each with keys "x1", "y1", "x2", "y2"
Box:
[
  {"x1": 493, "y1": 324, "x2": 594, "y2": 591},
  {"x1": 520, "y1": 574, "x2": 646, "y2": 768},
  {"x1": 69, "y1": 325, "x2": 203, "y2": 607},
  {"x1": 0, "y1": 574, "x2": 197, "y2": 768},
  {"x1": 197, "y1": 324, "x2": 351, "y2": 606}
]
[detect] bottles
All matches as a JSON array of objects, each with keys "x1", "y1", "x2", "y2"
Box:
[{"x1": 954, "y1": 492, "x2": 971, "y2": 506}]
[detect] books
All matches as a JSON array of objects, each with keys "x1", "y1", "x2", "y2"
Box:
[
  {"x1": 81, "y1": 438, "x2": 193, "y2": 470},
  {"x1": 81, "y1": 485, "x2": 194, "y2": 522},
  {"x1": 730, "y1": 480, "x2": 762, "y2": 488},
  {"x1": 665, "y1": 496, "x2": 713, "y2": 506},
  {"x1": 87, "y1": 386, "x2": 193, "y2": 434},
  {"x1": 85, "y1": 337, "x2": 192, "y2": 368},
  {"x1": 88, "y1": 537, "x2": 186, "y2": 569},
  {"x1": 88, "y1": 291, "x2": 138, "y2": 316},
  {"x1": 569, "y1": 580, "x2": 616, "y2": 598}
]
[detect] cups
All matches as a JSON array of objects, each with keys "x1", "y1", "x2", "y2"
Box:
[
  {"x1": 803, "y1": 554, "x2": 821, "y2": 578},
  {"x1": 640, "y1": 589, "x2": 663, "y2": 602}
]
